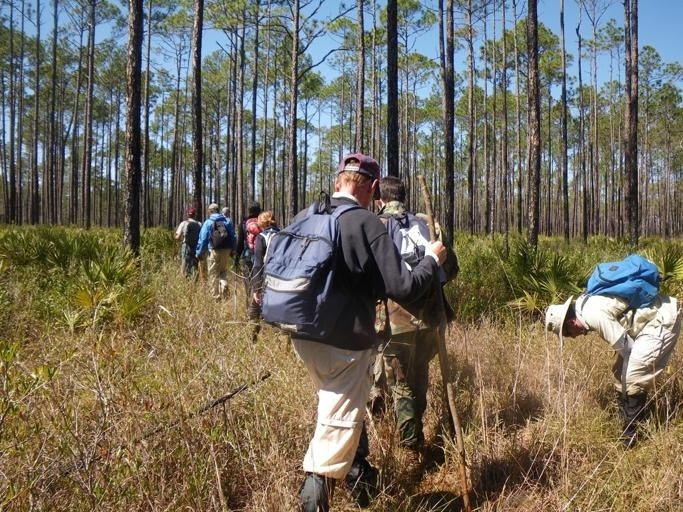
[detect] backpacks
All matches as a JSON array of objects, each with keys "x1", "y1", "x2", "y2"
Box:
[
  {"x1": 263, "y1": 201, "x2": 368, "y2": 337},
  {"x1": 211, "y1": 218, "x2": 235, "y2": 249},
  {"x1": 185, "y1": 221, "x2": 201, "y2": 252},
  {"x1": 382, "y1": 211, "x2": 445, "y2": 280},
  {"x1": 246, "y1": 216, "x2": 259, "y2": 253},
  {"x1": 263, "y1": 233, "x2": 276, "y2": 247},
  {"x1": 579, "y1": 254, "x2": 661, "y2": 313}
]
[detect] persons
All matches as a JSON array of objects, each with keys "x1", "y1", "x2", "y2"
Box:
[
  {"x1": 194, "y1": 203, "x2": 238, "y2": 305},
  {"x1": 174, "y1": 207, "x2": 203, "y2": 287},
  {"x1": 220, "y1": 207, "x2": 234, "y2": 227},
  {"x1": 290, "y1": 152, "x2": 448, "y2": 511},
  {"x1": 545, "y1": 293, "x2": 682, "y2": 451},
  {"x1": 232, "y1": 201, "x2": 263, "y2": 310},
  {"x1": 246, "y1": 210, "x2": 282, "y2": 343},
  {"x1": 366, "y1": 176, "x2": 459, "y2": 456},
  {"x1": 366, "y1": 341, "x2": 393, "y2": 418}
]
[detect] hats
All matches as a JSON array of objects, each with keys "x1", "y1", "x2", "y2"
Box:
[
  {"x1": 335, "y1": 154, "x2": 381, "y2": 200},
  {"x1": 187, "y1": 206, "x2": 200, "y2": 216},
  {"x1": 543, "y1": 295, "x2": 574, "y2": 352}
]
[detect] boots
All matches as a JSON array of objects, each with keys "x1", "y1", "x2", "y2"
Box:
[
  {"x1": 298, "y1": 472, "x2": 336, "y2": 512},
  {"x1": 347, "y1": 435, "x2": 380, "y2": 490},
  {"x1": 616, "y1": 390, "x2": 646, "y2": 448}
]
